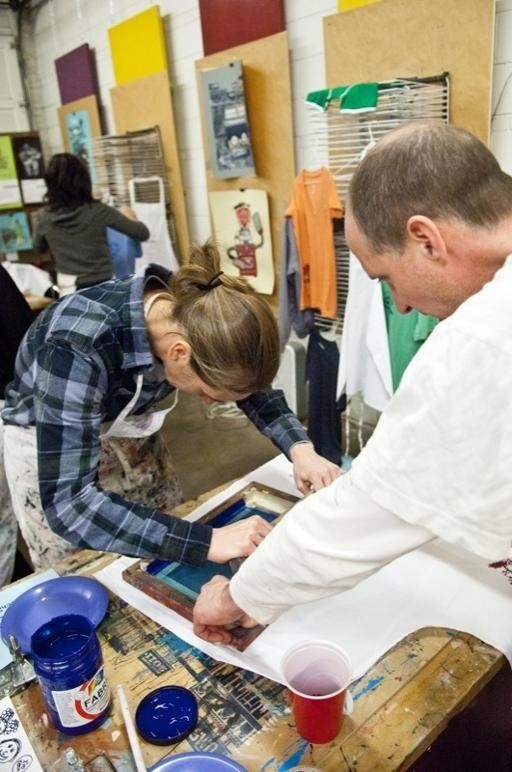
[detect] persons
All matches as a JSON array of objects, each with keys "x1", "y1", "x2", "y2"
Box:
[
  {"x1": 31, "y1": 153, "x2": 149, "y2": 297},
  {"x1": 2, "y1": 258, "x2": 342, "y2": 576},
  {"x1": 194, "y1": 123, "x2": 512, "y2": 644}
]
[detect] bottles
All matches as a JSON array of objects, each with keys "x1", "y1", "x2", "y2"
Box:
[{"x1": 30, "y1": 612, "x2": 112, "y2": 735}]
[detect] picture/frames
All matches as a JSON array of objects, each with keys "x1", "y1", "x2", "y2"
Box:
[{"x1": 122, "y1": 481, "x2": 302, "y2": 652}]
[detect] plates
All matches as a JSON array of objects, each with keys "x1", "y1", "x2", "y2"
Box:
[
  {"x1": 1, "y1": 575, "x2": 109, "y2": 657},
  {"x1": 147, "y1": 752, "x2": 247, "y2": 772}
]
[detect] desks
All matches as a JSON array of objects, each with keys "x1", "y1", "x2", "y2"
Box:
[{"x1": 1, "y1": 470, "x2": 508, "y2": 772}]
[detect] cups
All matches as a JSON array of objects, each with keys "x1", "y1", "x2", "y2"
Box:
[{"x1": 282, "y1": 640, "x2": 350, "y2": 744}]
